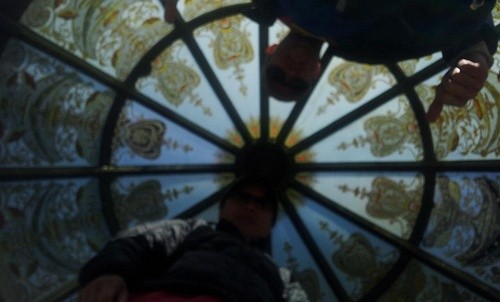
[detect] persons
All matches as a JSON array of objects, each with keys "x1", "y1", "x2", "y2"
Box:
[
  {"x1": 73, "y1": 168, "x2": 289, "y2": 302},
  {"x1": 249, "y1": 0, "x2": 497, "y2": 123}
]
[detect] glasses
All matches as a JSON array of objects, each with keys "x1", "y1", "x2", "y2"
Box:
[
  {"x1": 224, "y1": 190, "x2": 276, "y2": 214},
  {"x1": 264, "y1": 60, "x2": 315, "y2": 95}
]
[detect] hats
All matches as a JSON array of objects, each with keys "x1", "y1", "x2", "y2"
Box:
[{"x1": 217, "y1": 175, "x2": 280, "y2": 225}]
